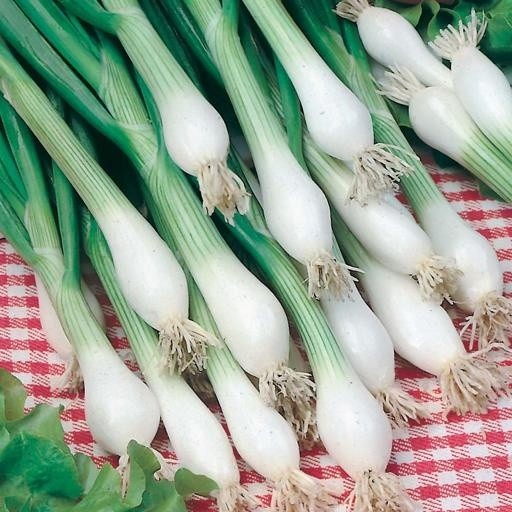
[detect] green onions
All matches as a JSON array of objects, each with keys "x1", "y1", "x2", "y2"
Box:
[{"x1": 0, "y1": 0, "x2": 512, "y2": 512}]
